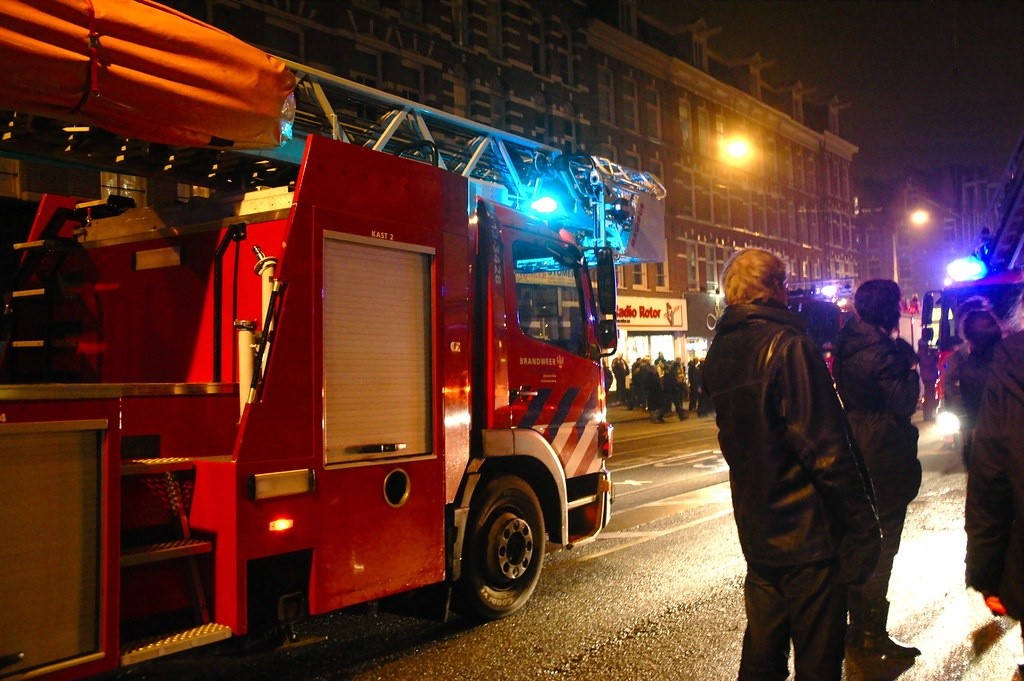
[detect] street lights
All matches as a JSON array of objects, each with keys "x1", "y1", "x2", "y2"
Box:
[{"x1": 892, "y1": 208, "x2": 928, "y2": 285}]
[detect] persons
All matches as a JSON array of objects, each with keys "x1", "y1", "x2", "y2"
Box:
[
  {"x1": 963, "y1": 288, "x2": 1024, "y2": 681},
  {"x1": 704, "y1": 248, "x2": 883, "y2": 681},
  {"x1": 603, "y1": 352, "x2": 714, "y2": 423},
  {"x1": 821, "y1": 278, "x2": 1020, "y2": 658},
  {"x1": 972, "y1": 227, "x2": 998, "y2": 277}
]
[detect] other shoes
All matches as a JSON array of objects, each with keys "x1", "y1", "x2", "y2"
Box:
[
  {"x1": 984, "y1": 597, "x2": 1007, "y2": 617},
  {"x1": 658, "y1": 418, "x2": 665, "y2": 423},
  {"x1": 681, "y1": 417, "x2": 687, "y2": 421},
  {"x1": 924, "y1": 417, "x2": 935, "y2": 421}
]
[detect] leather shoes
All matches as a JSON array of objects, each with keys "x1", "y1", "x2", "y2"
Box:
[{"x1": 846, "y1": 623, "x2": 920, "y2": 658}]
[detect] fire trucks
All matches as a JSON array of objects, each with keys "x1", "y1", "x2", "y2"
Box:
[
  {"x1": 920, "y1": 137, "x2": 1024, "y2": 419},
  {"x1": 0, "y1": 1, "x2": 667, "y2": 680}
]
